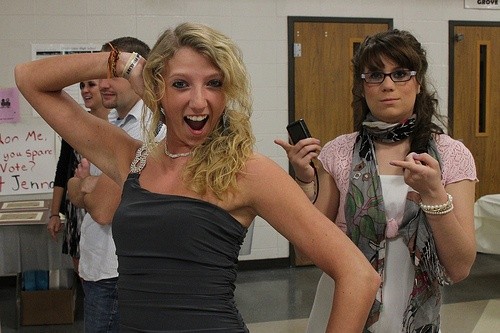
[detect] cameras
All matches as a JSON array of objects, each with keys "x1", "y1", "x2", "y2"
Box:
[{"x1": 287, "y1": 119, "x2": 315, "y2": 155}]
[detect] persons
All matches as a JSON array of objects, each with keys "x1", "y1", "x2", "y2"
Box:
[
  {"x1": 67, "y1": 36, "x2": 168, "y2": 332},
  {"x1": 273, "y1": 28, "x2": 478, "y2": 332},
  {"x1": 14, "y1": 22, "x2": 380, "y2": 333},
  {"x1": 46, "y1": 77, "x2": 112, "y2": 282}
]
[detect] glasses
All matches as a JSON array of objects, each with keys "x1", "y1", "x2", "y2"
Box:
[{"x1": 361, "y1": 70, "x2": 417, "y2": 84}]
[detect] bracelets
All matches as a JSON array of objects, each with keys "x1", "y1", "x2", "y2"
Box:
[
  {"x1": 49, "y1": 214, "x2": 60, "y2": 218},
  {"x1": 105, "y1": 40, "x2": 120, "y2": 78},
  {"x1": 120, "y1": 50, "x2": 142, "y2": 79},
  {"x1": 295, "y1": 175, "x2": 313, "y2": 183},
  {"x1": 418, "y1": 192, "x2": 453, "y2": 215}
]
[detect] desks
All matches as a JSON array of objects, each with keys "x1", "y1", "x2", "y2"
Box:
[{"x1": 0, "y1": 200, "x2": 68, "y2": 289}]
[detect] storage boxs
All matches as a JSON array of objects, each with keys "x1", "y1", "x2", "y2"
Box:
[{"x1": 20, "y1": 274, "x2": 78, "y2": 327}]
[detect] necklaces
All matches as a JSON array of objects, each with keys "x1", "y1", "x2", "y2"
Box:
[{"x1": 163, "y1": 133, "x2": 198, "y2": 158}]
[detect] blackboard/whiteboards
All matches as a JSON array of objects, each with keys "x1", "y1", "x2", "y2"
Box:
[{"x1": 0, "y1": 115, "x2": 65, "y2": 200}]
